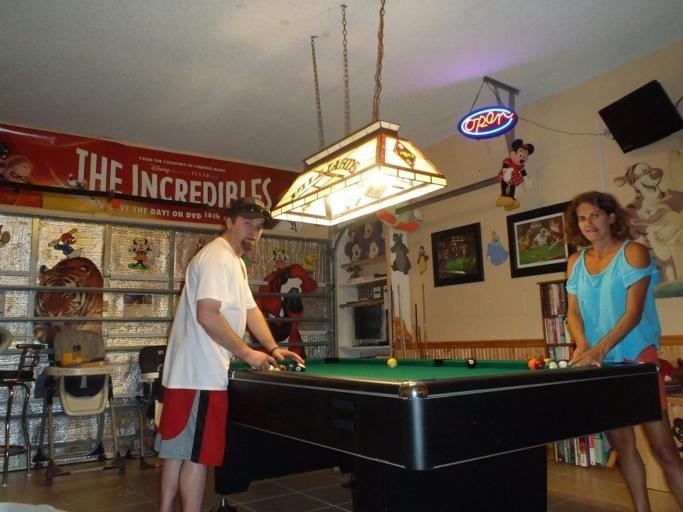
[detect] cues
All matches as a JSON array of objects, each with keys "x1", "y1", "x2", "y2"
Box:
[{"x1": 389, "y1": 283, "x2": 428, "y2": 360}]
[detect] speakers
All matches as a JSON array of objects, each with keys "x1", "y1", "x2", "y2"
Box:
[{"x1": 598, "y1": 80, "x2": 683, "y2": 153}]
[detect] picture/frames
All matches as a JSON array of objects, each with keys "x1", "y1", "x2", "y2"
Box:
[
  {"x1": 507, "y1": 198, "x2": 579, "y2": 280},
  {"x1": 429, "y1": 221, "x2": 486, "y2": 289}
]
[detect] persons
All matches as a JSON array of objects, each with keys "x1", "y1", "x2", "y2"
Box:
[
  {"x1": 562, "y1": 190, "x2": 681, "y2": 511},
  {"x1": 150, "y1": 195, "x2": 305, "y2": 512}
]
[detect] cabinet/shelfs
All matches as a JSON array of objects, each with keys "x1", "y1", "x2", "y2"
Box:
[
  {"x1": 634, "y1": 390, "x2": 682, "y2": 493},
  {"x1": 537, "y1": 278, "x2": 614, "y2": 470}
]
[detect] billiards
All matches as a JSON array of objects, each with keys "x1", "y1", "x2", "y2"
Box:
[
  {"x1": 251, "y1": 365, "x2": 256, "y2": 370},
  {"x1": 268, "y1": 362, "x2": 306, "y2": 372},
  {"x1": 387, "y1": 358, "x2": 397, "y2": 368},
  {"x1": 528, "y1": 357, "x2": 567, "y2": 369},
  {"x1": 466, "y1": 358, "x2": 476, "y2": 369}
]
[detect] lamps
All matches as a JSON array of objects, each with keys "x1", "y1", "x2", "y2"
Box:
[{"x1": 270, "y1": 0, "x2": 447, "y2": 227}]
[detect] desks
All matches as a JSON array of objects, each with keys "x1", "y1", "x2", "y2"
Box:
[{"x1": 220, "y1": 356, "x2": 666, "y2": 512}]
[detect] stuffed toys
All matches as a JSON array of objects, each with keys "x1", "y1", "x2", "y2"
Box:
[{"x1": 494, "y1": 139, "x2": 536, "y2": 212}]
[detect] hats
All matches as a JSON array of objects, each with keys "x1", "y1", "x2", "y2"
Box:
[{"x1": 224, "y1": 197, "x2": 279, "y2": 229}]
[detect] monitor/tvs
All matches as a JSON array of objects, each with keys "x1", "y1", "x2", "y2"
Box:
[{"x1": 355, "y1": 304, "x2": 382, "y2": 339}]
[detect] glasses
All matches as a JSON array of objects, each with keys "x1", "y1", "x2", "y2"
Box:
[{"x1": 240, "y1": 202, "x2": 271, "y2": 217}]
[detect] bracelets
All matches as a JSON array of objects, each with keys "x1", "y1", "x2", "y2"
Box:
[{"x1": 266, "y1": 345, "x2": 281, "y2": 355}]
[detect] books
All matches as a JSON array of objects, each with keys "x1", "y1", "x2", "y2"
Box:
[
  {"x1": 553, "y1": 432, "x2": 612, "y2": 468},
  {"x1": 543, "y1": 280, "x2": 574, "y2": 361}
]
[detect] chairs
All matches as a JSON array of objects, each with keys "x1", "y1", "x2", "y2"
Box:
[{"x1": 0, "y1": 328, "x2": 175, "y2": 487}]
